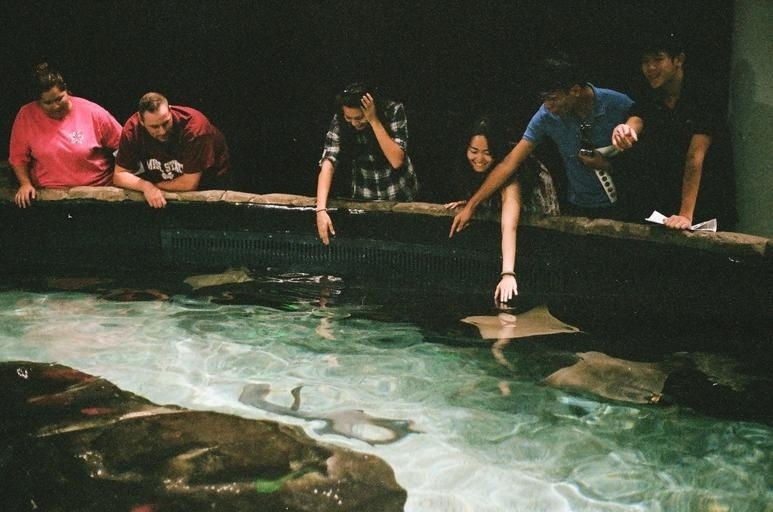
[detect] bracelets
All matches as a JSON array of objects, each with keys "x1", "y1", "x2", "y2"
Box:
[
  {"x1": 500, "y1": 271, "x2": 518, "y2": 277},
  {"x1": 313, "y1": 208, "x2": 329, "y2": 215}
]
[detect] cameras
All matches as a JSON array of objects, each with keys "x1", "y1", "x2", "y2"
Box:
[{"x1": 576, "y1": 123, "x2": 593, "y2": 156}]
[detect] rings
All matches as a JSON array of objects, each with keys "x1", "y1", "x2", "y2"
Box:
[{"x1": 614, "y1": 133, "x2": 619, "y2": 140}]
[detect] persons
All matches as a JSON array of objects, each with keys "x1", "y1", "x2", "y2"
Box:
[
  {"x1": 445, "y1": 55, "x2": 635, "y2": 239},
  {"x1": 313, "y1": 80, "x2": 420, "y2": 248},
  {"x1": 609, "y1": 29, "x2": 737, "y2": 236},
  {"x1": 6, "y1": 61, "x2": 125, "y2": 211},
  {"x1": 110, "y1": 93, "x2": 232, "y2": 210},
  {"x1": 445, "y1": 117, "x2": 561, "y2": 304}
]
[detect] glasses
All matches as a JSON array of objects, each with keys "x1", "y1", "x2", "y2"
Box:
[{"x1": 578, "y1": 122, "x2": 594, "y2": 156}]
